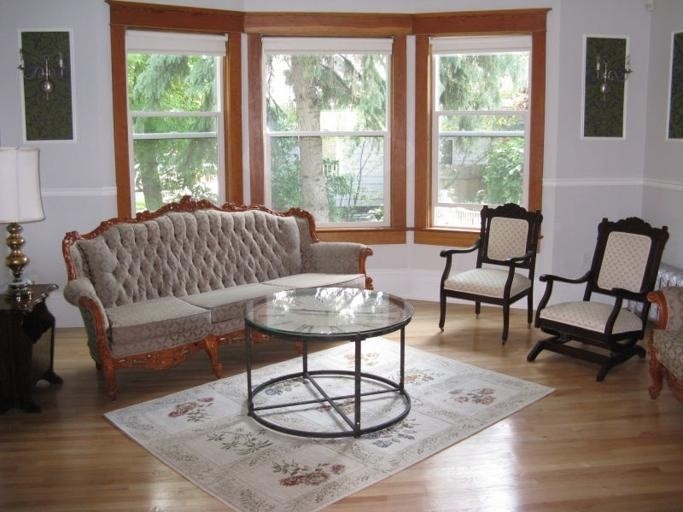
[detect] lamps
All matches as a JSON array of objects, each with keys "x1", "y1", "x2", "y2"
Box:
[
  {"x1": 19, "y1": 48, "x2": 68, "y2": 101},
  {"x1": 1, "y1": 147, "x2": 46, "y2": 300},
  {"x1": 590, "y1": 57, "x2": 632, "y2": 103}
]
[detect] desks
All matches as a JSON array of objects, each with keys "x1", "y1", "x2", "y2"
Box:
[
  {"x1": 103, "y1": 336, "x2": 557, "y2": 512},
  {"x1": 0, "y1": 284, "x2": 62, "y2": 413}
]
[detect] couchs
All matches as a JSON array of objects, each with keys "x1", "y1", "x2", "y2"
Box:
[{"x1": 63, "y1": 195, "x2": 374, "y2": 403}]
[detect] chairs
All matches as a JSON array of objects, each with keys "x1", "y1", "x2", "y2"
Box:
[
  {"x1": 644, "y1": 287, "x2": 683, "y2": 406},
  {"x1": 439, "y1": 202, "x2": 544, "y2": 344},
  {"x1": 528, "y1": 216, "x2": 669, "y2": 381}
]
